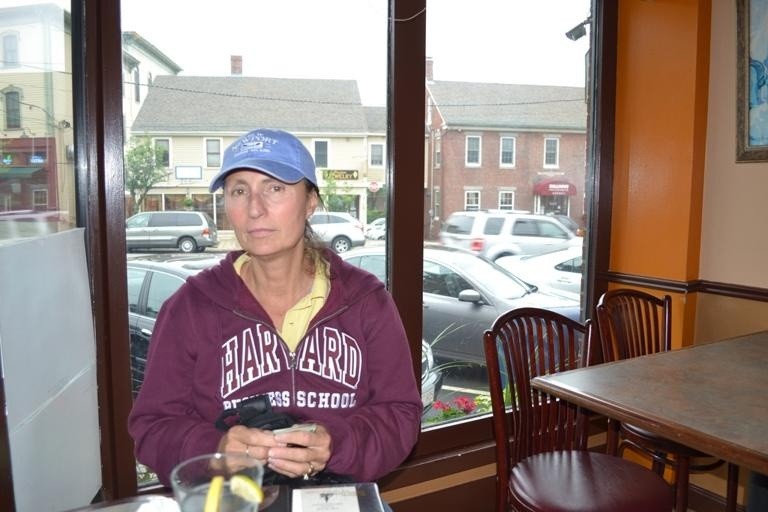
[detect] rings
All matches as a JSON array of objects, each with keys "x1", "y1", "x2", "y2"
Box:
[
  {"x1": 246, "y1": 445, "x2": 249, "y2": 458},
  {"x1": 303, "y1": 462, "x2": 314, "y2": 477}
]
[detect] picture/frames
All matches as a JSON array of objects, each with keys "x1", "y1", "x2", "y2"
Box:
[{"x1": 735, "y1": 0, "x2": 768, "y2": 164}]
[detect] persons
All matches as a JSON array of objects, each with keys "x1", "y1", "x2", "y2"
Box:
[{"x1": 126, "y1": 128, "x2": 423, "y2": 483}]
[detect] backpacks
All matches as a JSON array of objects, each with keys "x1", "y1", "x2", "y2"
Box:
[{"x1": 214, "y1": 395, "x2": 346, "y2": 485}]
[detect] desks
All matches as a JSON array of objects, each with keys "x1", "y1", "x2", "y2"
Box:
[{"x1": 531, "y1": 331, "x2": 768, "y2": 512}]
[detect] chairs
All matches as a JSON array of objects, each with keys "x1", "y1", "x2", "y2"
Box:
[
  {"x1": 598, "y1": 288, "x2": 739, "y2": 512},
  {"x1": 482, "y1": 307, "x2": 674, "y2": 512}
]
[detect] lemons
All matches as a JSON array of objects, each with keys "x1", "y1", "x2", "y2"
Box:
[
  {"x1": 229, "y1": 475, "x2": 265, "y2": 505},
  {"x1": 202, "y1": 475, "x2": 225, "y2": 512}
]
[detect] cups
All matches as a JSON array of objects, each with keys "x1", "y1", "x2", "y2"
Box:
[{"x1": 167, "y1": 451, "x2": 266, "y2": 512}]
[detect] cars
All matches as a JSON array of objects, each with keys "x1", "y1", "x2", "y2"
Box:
[{"x1": 126, "y1": 251, "x2": 442, "y2": 417}]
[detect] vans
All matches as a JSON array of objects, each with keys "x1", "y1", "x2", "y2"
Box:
[{"x1": 124, "y1": 210, "x2": 218, "y2": 253}]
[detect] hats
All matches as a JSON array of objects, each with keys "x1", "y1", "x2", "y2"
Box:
[{"x1": 209, "y1": 130, "x2": 319, "y2": 194}]
